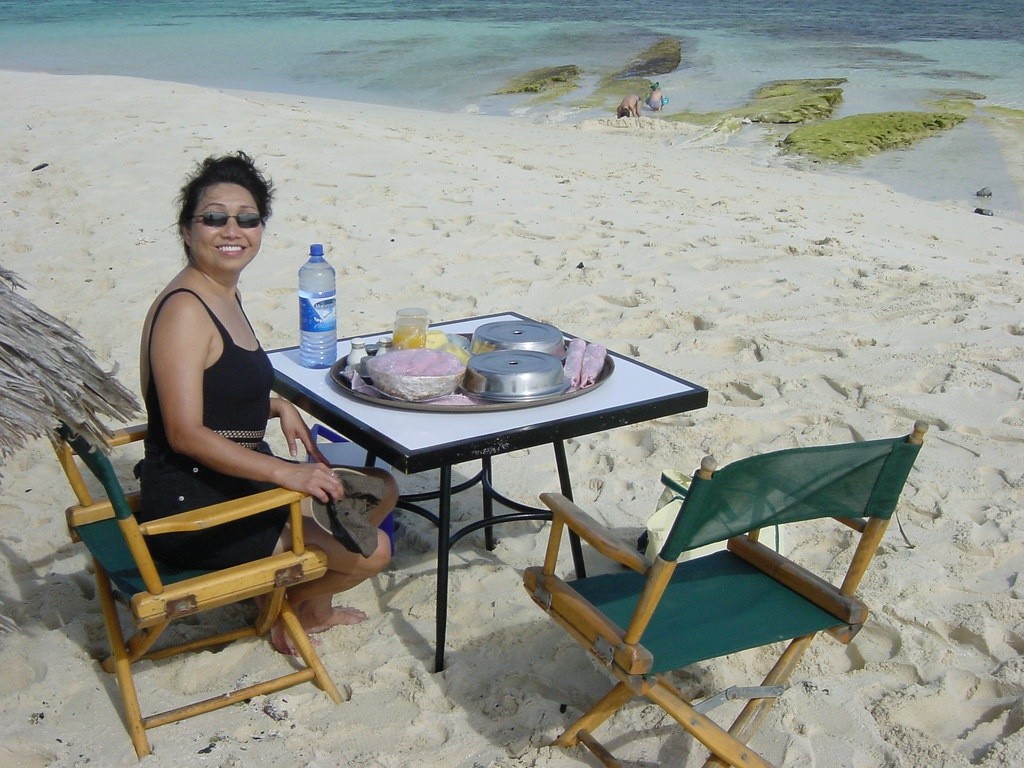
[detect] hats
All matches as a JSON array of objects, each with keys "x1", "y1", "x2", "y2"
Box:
[{"x1": 309, "y1": 468, "x2": 384, "y2": 558}]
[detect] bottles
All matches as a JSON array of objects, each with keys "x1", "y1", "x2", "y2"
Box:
[
  {"x1": 375, "y1": 338, "x2": 394, "y2": 356},
  {"x1": 298, "y1": 243, "x2": 337, "y2": 368},
  {"x1": 347, "y1": 338, "x2": 368, "y2": 370}
]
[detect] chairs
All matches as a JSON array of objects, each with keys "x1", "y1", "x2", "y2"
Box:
[
  {"x1": 45, "y1": 417, "x2": 344, "y2": 759},
  {"x1": 523, "y1": 419, "x2": 929, "y2": 768}
]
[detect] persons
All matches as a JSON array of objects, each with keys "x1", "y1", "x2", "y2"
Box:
[
  {"x1": 132, "y1": 149, "x2": 399, "y2": 657},
  {"x1": 646, "y1": 86, "x2": 664, "y2": 113},
  {"x1": 617, "y1": 94, "x2": 641, "y2": 119}
]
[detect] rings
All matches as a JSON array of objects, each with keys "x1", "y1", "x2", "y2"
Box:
[{"x1": 330, "y1": 472, "x2": 334, "y2": 477}]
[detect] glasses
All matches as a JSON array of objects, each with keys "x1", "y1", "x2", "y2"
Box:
[{"x1": 191, "y1": 211, "x2": 262, "y2": 229}]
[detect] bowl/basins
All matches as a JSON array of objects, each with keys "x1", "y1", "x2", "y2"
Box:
[{"x1": 366, "y1": 354, "x2": 467, "y2": 400}]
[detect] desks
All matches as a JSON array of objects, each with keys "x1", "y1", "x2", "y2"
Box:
[{"x1": 264, "y1": 311, "x2": 709, "y2": 673}]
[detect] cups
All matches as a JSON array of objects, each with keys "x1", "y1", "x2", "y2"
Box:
[
  {"x1": 392, "y1": 316, "x2": 427, "y2": 348},
  {"x1": 395, "y1": 307, "x2": 427, "y2": 328}
]
